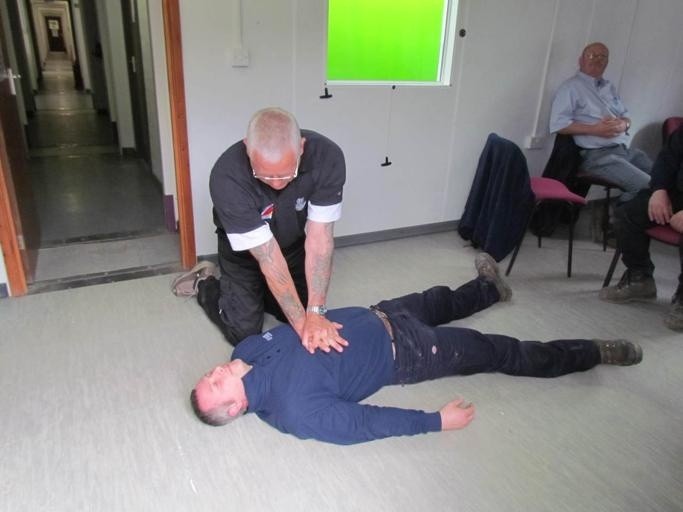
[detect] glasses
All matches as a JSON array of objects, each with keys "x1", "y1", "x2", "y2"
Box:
[
  {"x1": 583, "y1": 52, "x2": 608, "y2": 60},
  {"x1": 249, "y1": 156, "x2": 299, "y2": 181}
]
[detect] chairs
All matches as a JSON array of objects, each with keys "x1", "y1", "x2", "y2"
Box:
[
  {"x1": 486, "y1": 133, "x2": 586, "y2": 278},
  {"x1": 661, "y1": 116, "x2": 681, "y2": 143},
  {"x1": 601, "y1": 221, "x2": 682, "y2": 306},
  {"x1": 577, "y1": 177, "x2": 626, "y2": 250}
]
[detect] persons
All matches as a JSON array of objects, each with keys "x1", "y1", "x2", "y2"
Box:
[
  {"x1": 188, "y1": 251, "x2": 641, "y2": 444},
  {"x1": 599, "y1": 119, "x2": 683, "y2": 331},
  {"x1": 548, "y1": 41, "x2": 655, "y2": 230},
  {"x1": 170, "y1": 106, "x2": 347, "y2": 355}
]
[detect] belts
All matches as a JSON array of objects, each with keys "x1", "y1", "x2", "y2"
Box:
[{"x1": 365, "y1": 306, "x2": 396, "y2": 361}]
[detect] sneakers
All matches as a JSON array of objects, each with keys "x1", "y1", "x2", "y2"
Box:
[
  {"x1": 598, "y1": 270, "x2": 657, "y2": 304},
  {"x1": 171, "y1": 261, "x2": 221, "y2": 297},
  {"x1": 474, "y1": 252, "x2": 512, "y2": 302},
  {"x1": 590, "y1": 337, "x2": 642, "y2": 366},
  {"x1": 664, "y1": 292, "x2": 682, "y2": 331}
]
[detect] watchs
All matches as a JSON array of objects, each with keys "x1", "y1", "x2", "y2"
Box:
[
  {"x1": 306, "y1": 304, "x2": 328, "y2": 314},
  {"x1": 621, "y1": 117, "x2": 629, "y2": 132}
]
